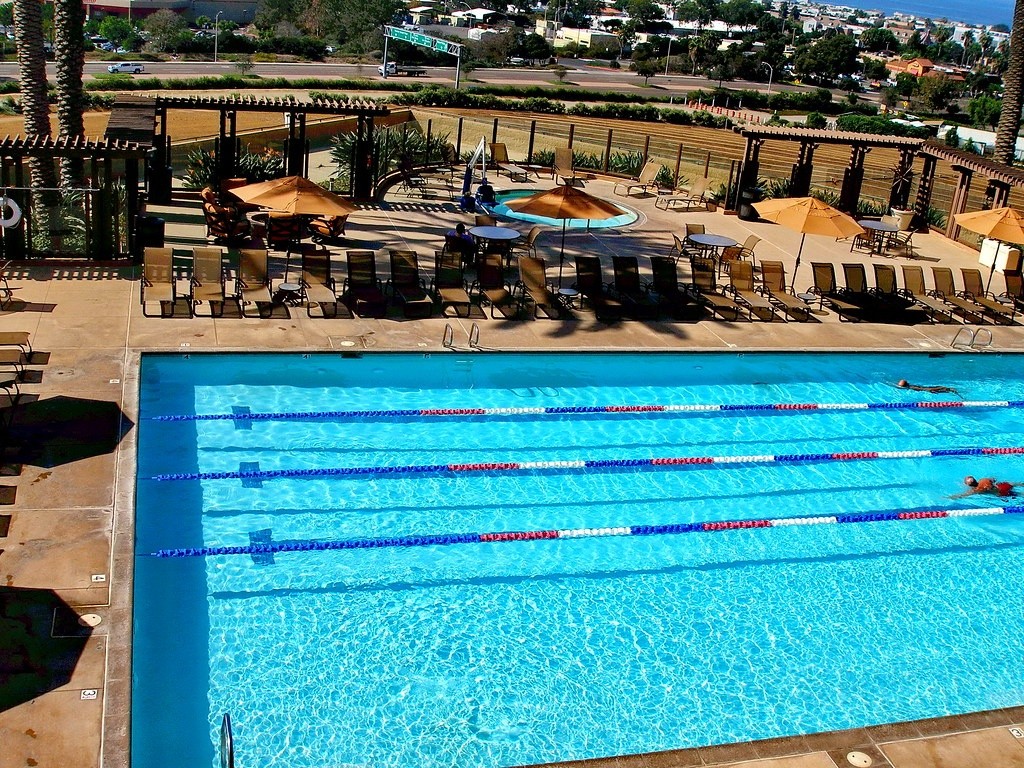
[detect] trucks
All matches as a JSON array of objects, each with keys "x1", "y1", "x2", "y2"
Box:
[{"x1": 378, "y1": 61, "x2": 428, "y2": 77}]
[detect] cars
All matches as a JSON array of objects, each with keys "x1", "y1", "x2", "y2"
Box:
[
  {"x1": 837, "y1": 71, "x2": 899, "y2": 93},
  {"x1": 91, "y1": 26, "x2": 176, "y2": 55}
]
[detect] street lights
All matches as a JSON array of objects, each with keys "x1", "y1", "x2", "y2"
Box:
[
  {"x1": 664, "y1": 37, "x2": 677, "y2": 77},
  {"x1": 762, "y1": 62, "x2": 773, "y2": 93},
  {"x1": 213, "y1": 10, "x2": 223, "y2": 62}
]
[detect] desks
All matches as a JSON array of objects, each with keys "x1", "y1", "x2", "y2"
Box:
[
  {"x1": 409, "y1": 178, "x2": 425, "y2": 194},
  {"x1": 528, "y1": 164, "x2": 543, "y2": 179},
  {"x1": 468, "y1": 226, "x2": 521, "y2": 269},
  {"x1": 278, "y1": 282, "x2": 304, "y2": 307},
  {"x1": 656, "y1": 189, "x2": 673, "y2": 205},
  {"x1": 688, "y1": 233, "x2": 738, "y2": 259},
  {"x1": 995, "y1": 296, "x2": 1012, "y2": 314},
  {"x1": 436, "y1": 168, "x2": 451, "y2": 181},
  {"x1": 857, "y1": 219, "x2": 900, "y2": 255},
  {"x1": 557, "y1": 288, "x2": 583, "y2": 309},
  {"x1": 796, "y1": 293, "x2": 818, "y2": 314}
]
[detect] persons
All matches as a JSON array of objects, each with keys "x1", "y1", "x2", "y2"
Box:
[
  {"x1": 949, "y1": 476, "x2": 1024, "y2": 500},
  {"x1": 898, "y1": 380, "x2": 962, "y2": 398},
  {"x1": 446, "y1": 175, "x2": 500, "y2": 268}
]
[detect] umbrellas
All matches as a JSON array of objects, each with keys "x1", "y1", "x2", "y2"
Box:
[
  {"x1": 227, "y1": 172, "x2": 362, "y2": 283},
  {"x1": 751, "y1": 195, "x2": 866, "y2": 296},
  {"x1": 952, "y1": 204, "x2": 1024, "y2": 298},
  {"x1": 503, "y1": 182, "x2": 629, "y2": 289}
]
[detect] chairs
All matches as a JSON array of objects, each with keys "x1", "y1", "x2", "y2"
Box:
[
  {"x1": 0, "y1": 373, "x2": 20, "y2": 408},
  {"x1": 0, "y1": 349, "x2": 24, "y2": 382},
  {"x1": 0, "y1": 332, "x2": 33, "y2": 362},
  {"x1": 0, "y1": 259, "x2": 23, "y2": 312},
  {"x1": 139, "y1": 144, "x2": 1024, "y2": 326}
]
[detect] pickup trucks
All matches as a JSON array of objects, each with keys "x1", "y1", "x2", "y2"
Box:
[{"x1": 107, "y1": 62, "x2": 145, "y2": 74}]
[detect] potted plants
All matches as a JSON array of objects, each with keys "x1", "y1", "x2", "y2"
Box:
[{"x1": 888, "y1": 163, "x2": 921, "y2": 231}]
